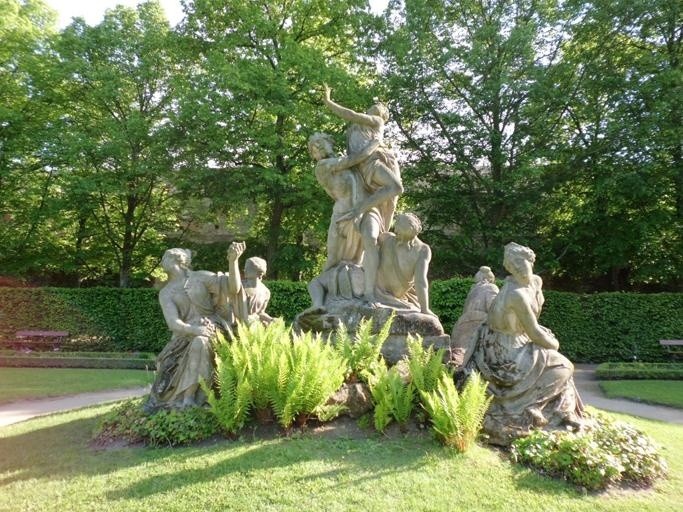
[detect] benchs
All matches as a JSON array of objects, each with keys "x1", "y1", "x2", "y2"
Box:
[
  {"x1": 2, "y1": 329, "x2": 70, "y2": 352},
  {"x1": 661, "y1": 339, "x2": 683, "y2": 361}
]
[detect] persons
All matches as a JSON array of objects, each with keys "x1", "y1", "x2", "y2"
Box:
[
  {"x1": 241, "y1": 256, "x2": 276, "y2": 323},
  {"x1": 473, "y1": 241, "x2": 590, "y2": 433},
  {"x1": 322, "y1": 82, "x2": 405, "y2": 235},
  {"x1": 296, "y1": 212, "x2": 441, "y2": 319},
  {"x1": 144, "y1": 241, "x2": 247, "y2": 416},
  {"x1": 450, "y1": 267, "x2": 499, "y2": 373},
  {"x1": 307, "y1": 132, "x2": 383, "y2": 310}
]
